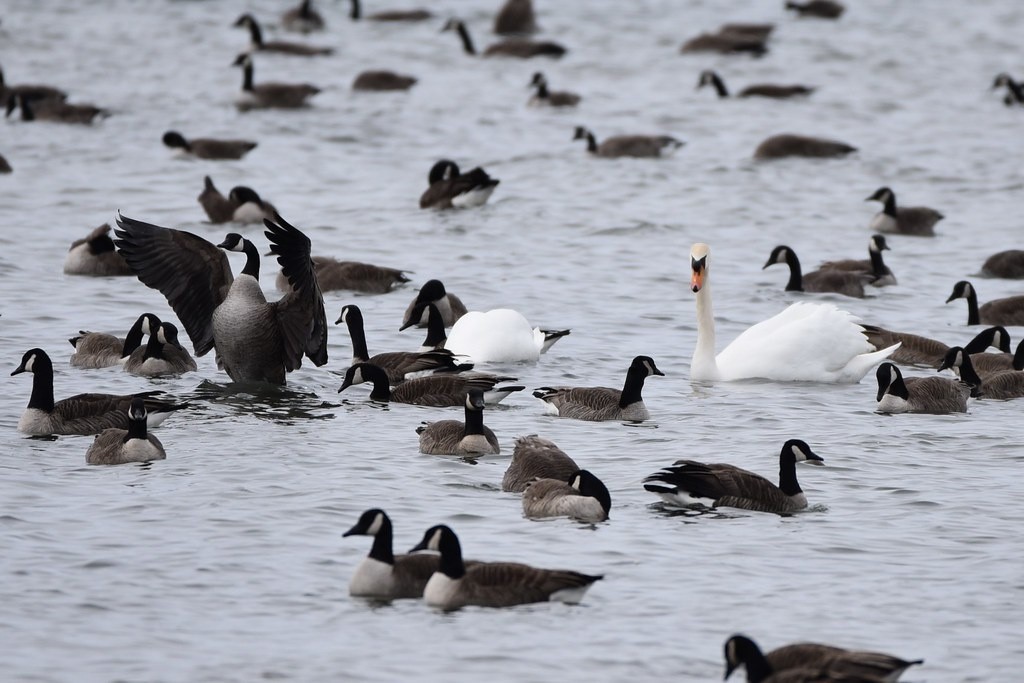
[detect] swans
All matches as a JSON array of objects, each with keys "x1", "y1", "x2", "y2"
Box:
[{"x1": 688, "y1": 243, "x2": 903, "y2": 384}]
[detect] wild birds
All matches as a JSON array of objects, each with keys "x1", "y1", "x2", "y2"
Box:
[
  {"x1": 415, "y1": 389, "x2": 500, "y2": 456},
  {"x1": 199, "y1": 174, "x2": 278, "y2": 225},
  {"x1": 228, "y1": 0, "x2": 1024, "y2": 115},
  {"x1": 11, "y1": 348, "x2": 190, "y2": 436},
  {"x1": 864, "y1": 186, "x2": 945, "y2": 237},
  {"x1": 420, "y1": 160, "x2": 499, "y2": 211},
  {"x1": 569, "y1": 126, "x2": 686, "y2": 161},
  {"x1": 67, "y1": 313, "x2": 198, "y2": 376},
  {"x1": 340, "y1": 363, "x2": 525, "y2": 408},
  {"x1": 857, "y1": 249, "x2": 1024, "y2": 401},
  {"x1": 753, "y1": 135, "x2": 857, "y2": 162},
  {"x1": 163, "y1": 130, "x2": 257, "y2": 161},
  {"x1": 86, "y1": 398, "x2": 166, "y2": 464},
  {"x1": 408, "y1": 524, "x2": 604, "y2": 612},
  {"x1": 263, "y1": 246, "x2": 415, "y2": 299},
  {"x1": 335, "y1": 304, "x2": 474, "y2": 385},
  {"x1": 341, "y1": 508, "x2": 486, "y2": 598},
  {"x1": 532, "y1": 355, "x2": 665, "y2": 421},
  {"x1": 503, "y1": 433, "x2": 612, "y2": 524},
  {"x1": 816, "y1": 234, "x2": 897, "y2": 288},
  {"x1": 724, "y1": 635, "x2": 924, "y2": 683},
  {"x1": 641, "y1": 437, "x2": 825, "y2": 522},
  {"x1": 874, "y1": 361, "x2": 977, "y2": 415},
  {"x1": 398, "y1": 279, "x2": 572, "y2": 355},
  {"x1": 0, "y1": 65, "x2": 114, "y2": 128},
  {"x1": 113, "y1": 208, "x2": 328, "y2": 387},
  {"x1": 761, "y1": 245, "x2": 882, "y2": 298}
]
